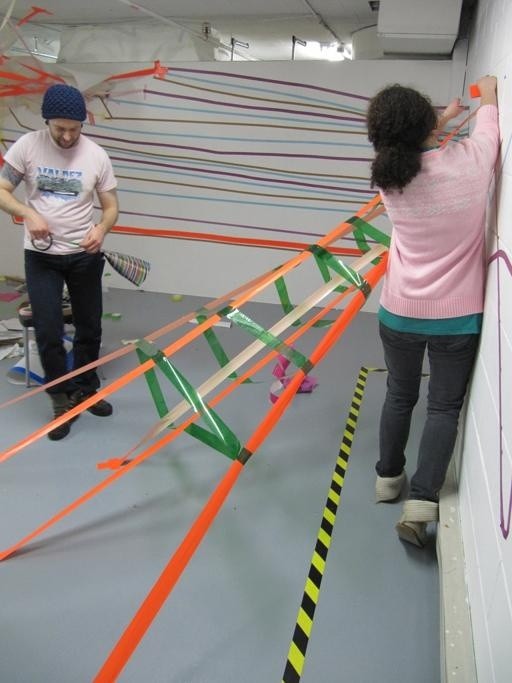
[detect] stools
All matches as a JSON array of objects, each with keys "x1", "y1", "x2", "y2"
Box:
[{"x1": 19, "y1": 300, "x2": 77, "y2": 390}]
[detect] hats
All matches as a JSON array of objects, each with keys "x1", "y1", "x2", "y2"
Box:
[{"x1": 42, "y1": 85, "x2": 86, "y2": 121}]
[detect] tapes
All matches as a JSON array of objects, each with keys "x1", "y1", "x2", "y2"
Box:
[{"x1": 32, "y1": 232, "x2": 53, "y2": 251}]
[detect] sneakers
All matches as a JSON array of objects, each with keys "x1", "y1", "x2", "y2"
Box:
[
  {"x1": 47, "y1": 390, "x2": 81, "y2": 440},
  {"x1": 81, "y1": 390, "x2": 112, "y2": 416},
  {"x1": 395, "y1": 515, "x2": 430, "y2": 548}
]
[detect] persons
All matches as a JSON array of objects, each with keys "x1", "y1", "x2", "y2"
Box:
[
  {"x1": 366, "y1": 75, "x2": 500, "y2": 549},
  {"x1": 0, "y1": 85, "x2": 120, "y2": 440}
]
